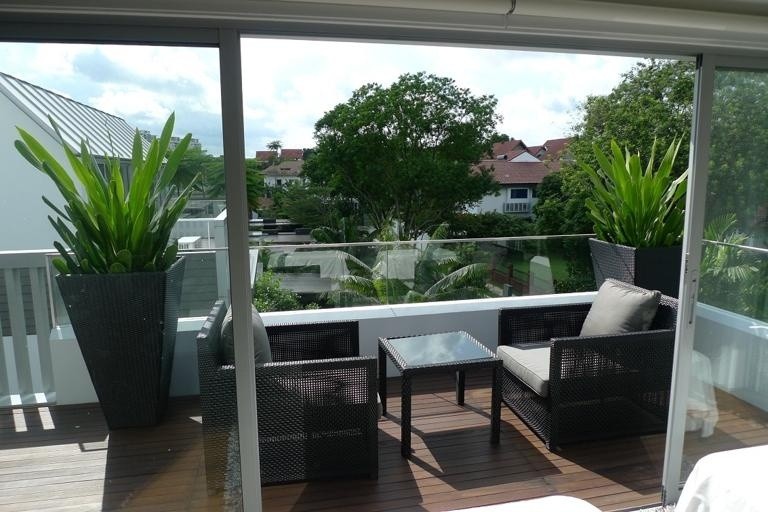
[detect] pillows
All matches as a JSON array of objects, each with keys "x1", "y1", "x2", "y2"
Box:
[
  {"x1": 575, "y1": 279, "x2": 662, "y2": 336},
  {"x1": 221, "y1": 299, "x2": 272, "y2": 365}
]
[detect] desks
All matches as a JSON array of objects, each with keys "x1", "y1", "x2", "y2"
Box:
[{"x1": 375, "y1": 328, "x2": 504, "y2": 460}]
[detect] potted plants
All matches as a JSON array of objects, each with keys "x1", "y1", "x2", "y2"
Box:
[
  {"x1": 568, "y1": 131, "x2": 693, "y2": 291},
  {"x1": 5, "y1": 110, "x2": 203, "y2": 431}
]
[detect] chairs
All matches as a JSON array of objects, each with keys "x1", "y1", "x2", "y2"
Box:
[
  {"x1": 195, "y1": 296, "x2": 379, "y2": 499},
  {"x1": 494, "y1": 296, "x2": 680, "y2": 457}
]
